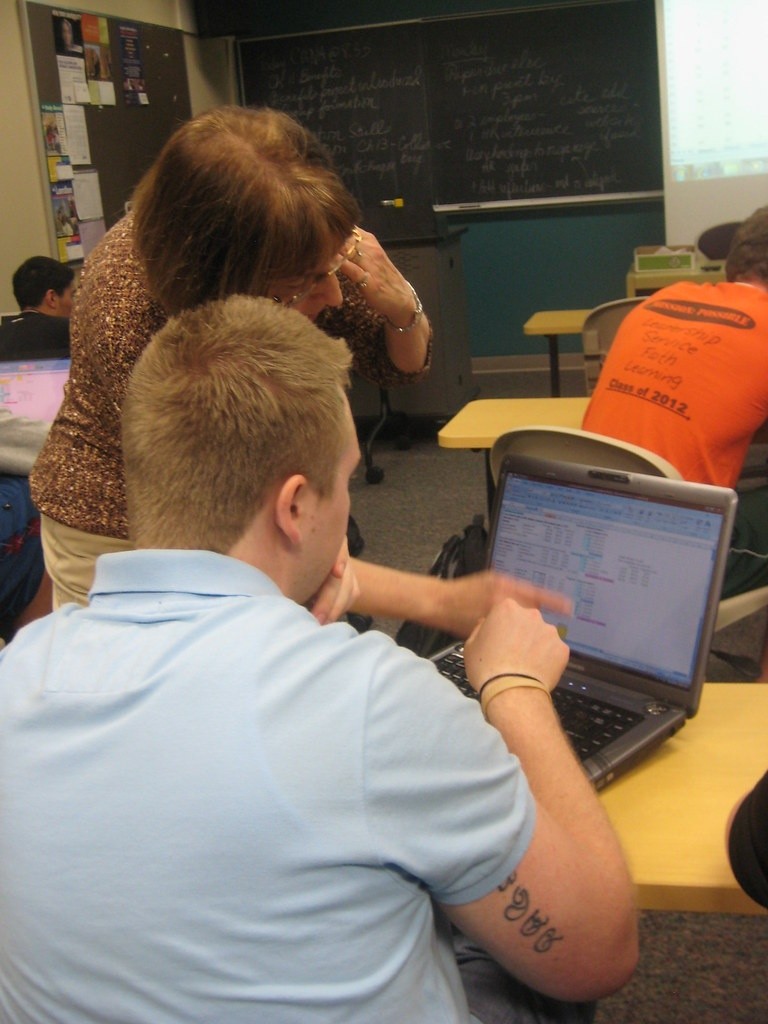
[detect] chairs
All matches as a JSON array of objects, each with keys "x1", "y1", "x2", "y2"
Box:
[
  {"x1": 581, "y1": 296, "x2": 650, "y2": 398},
  {"x1": 489, "y1": 425, "x2": 768, "y2": 682}
]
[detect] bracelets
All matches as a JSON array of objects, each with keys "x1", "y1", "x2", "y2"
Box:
[{"x1": 479, "y1": 672, "x2": 552, "y2": 716}]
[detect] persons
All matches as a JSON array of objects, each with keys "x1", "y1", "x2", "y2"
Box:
[
  {"x1": 725, "y1": 769, "x2": 768, "y2": 911},
  {"x1": 28, "y1": 107, "x2": 579, "y2": 646},
  {"x1": 0, "y1": 474, "x2": 49, "y2": 657},
  {"x1": 1, "y1": 293, "x2": 640, "y2": 1024},
  {"x1": 580, "y1": 200, "x2": 768, "y2": 682},
  {"x1": 0, "y1": 255, "x2": 77, "y2": 362}
]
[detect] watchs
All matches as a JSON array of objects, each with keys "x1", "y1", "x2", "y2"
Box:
[{"x1": 386, "y1": 282, "x2": 424, "y2": 335}]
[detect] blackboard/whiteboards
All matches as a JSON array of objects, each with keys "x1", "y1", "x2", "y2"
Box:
[{"x1": 235, "y1": 0, "x2": 664, "y2": 212}]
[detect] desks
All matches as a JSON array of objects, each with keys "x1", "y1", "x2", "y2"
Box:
[
  {"x1": 524, "y1": 309, "x2": 595, "y2": 398},
  {"x1": 438, "y1": 398, "x2": 592, "y2": 518},
  {"x1": 596, "y1": 682, "x2": 768, "y2": 915}
]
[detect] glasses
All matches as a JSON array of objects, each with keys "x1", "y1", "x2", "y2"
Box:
[{"x1": 262, "y1": 230, "x2": 362, "y2": 307}]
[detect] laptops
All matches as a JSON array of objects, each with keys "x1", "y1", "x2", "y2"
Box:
[
  {"x1": 0, "y1": 350, "x2": 72, "y2": 424},
  {"x1": 429, "y1": 455, "x2": 738, "y2": 792}
]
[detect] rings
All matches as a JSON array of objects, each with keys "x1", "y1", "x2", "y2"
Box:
[
  {"x1": 355, "y1": 272, "x2": 369, "y2": 288},
  {"x1": 351, "y1": 248, "x2": 363, "y2": 260}
]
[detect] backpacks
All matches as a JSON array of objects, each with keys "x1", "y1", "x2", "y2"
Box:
[{"x1": 394, "y1": 514, "x2": 491, "y2": 659}]
